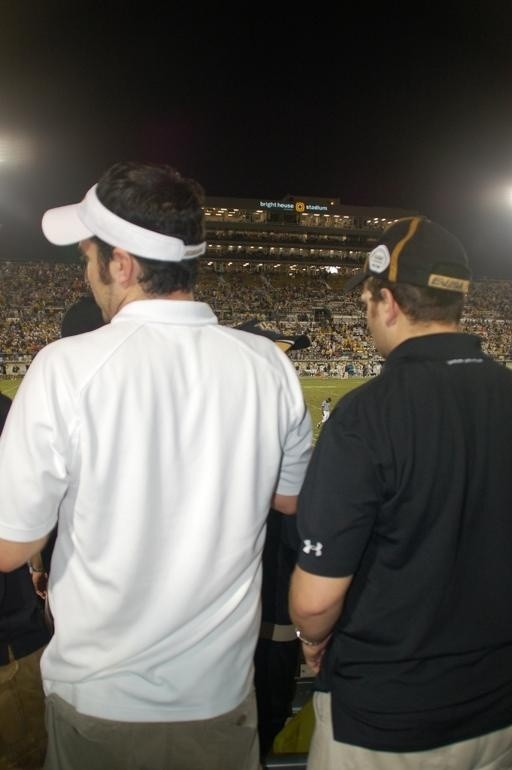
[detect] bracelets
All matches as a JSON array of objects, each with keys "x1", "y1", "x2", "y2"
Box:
[
  {"x1": 291, "y1": 626, "x2": 335, "y2": 646},
  {"x1": 28, "y1": 562, "x2": 46, "y2": 574}
]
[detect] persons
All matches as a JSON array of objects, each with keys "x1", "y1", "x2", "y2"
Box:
[
  {"x1": 0, "y1": 257, "x2": 511, "y2": 391},
  {"x1": 0, "y1": 394, "x2": 58, "y2": 770},
  {"x1": 252, "y1": 505, "x2": 303, "y2": 770},
  {"x1": 0, "y1": 160, "x2": 316, "y2": 770},
  {"x1": 316, "y1": 396, "x2": 333, "y2": 429},
  {"x1": 286, "y1": 215, "x2": 511, "y2": 770}
]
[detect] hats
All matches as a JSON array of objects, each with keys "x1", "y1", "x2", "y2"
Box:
[
  {"x1": 41, "y1": 183, "x2": 207, "y2": 262},
  {"x1": 230, "y1": 318, "x2": 311, "y2": 354},
  {"x1": 343, "y1": 214, "x2": 473, "y2": 295}
]
